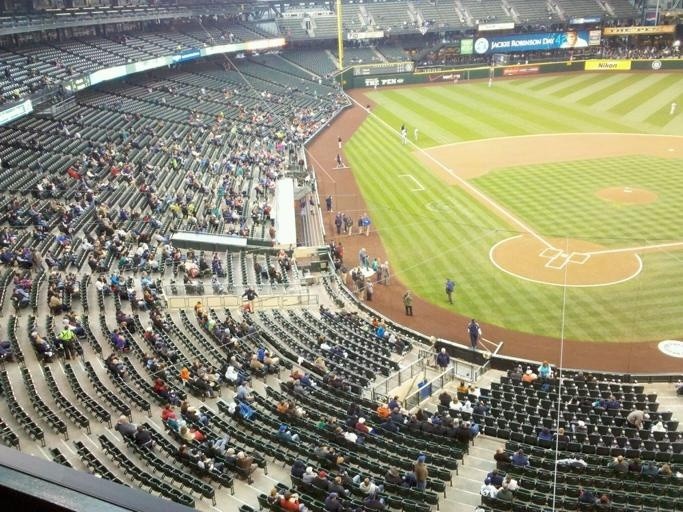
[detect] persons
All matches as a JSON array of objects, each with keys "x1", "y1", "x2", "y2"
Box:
[
  {"x1": 396, "y1": 124, "x2": 409, "y2": 147},
  {"x1": 399, "y1": 289, "x2": 415, "y2": 316},
  {"x1": 382, "y1": 316, "x2": 681, "y2": 510},
  {"x1": 444, "y1": 276, "x2": 457, "y2": 305},
  {"x1": 0, "y1": 1, "x2": 683, "y2": 116},
  {"x1": 0, "y1": 112, "x2": 383, "y2": 511},
  {"x1": 412, "y1": 128, "x2": 419, "y2": 143}
]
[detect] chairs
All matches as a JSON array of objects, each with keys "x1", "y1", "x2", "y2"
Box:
[{"x1": 1, "y1": 0, "x2": 683, "y2": 510}]
